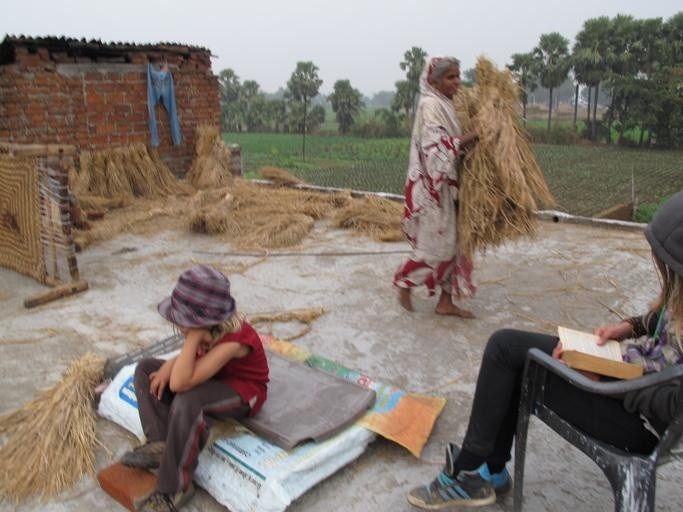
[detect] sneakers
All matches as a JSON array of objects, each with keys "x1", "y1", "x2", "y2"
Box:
[
  {"x1": 118, "y1": 439, "x2": 167, "y2": 468},
  {"x1": 137, "y1": 480, "x2": 196, "y2": 512},
  {"x1": 490, "y1": 455, "x2": 513, "y2": 495},
  {"x1": 403, "y1": 441, "x2": 498, "y2": 512}
]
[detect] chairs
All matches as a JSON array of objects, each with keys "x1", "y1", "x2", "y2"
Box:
[{"x1": 511, "y1": 346, "x2": 683, "y2": 511}]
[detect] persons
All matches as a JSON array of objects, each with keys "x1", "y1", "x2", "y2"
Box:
[
  {"x1": 389, "y1": 55, "x2": 484, "y2": 320},
  {"x1": 115, "y1": 263, "x2": 271, "y2": 512},
  {"x1": 406, "y1": 190, "x2": 682, "y2": 510}
]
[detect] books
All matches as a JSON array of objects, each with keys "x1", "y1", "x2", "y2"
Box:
[{"x1": 556, "y1": 322, "x2": 642, "y2": 383}]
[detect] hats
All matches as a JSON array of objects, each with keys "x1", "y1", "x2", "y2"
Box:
[
  {"x1": 642, "y1": 189, "x2": 683, "y2": 280},
  {"x1": 155, "y1": 264, "x2": 238, "y2": 332}
]
